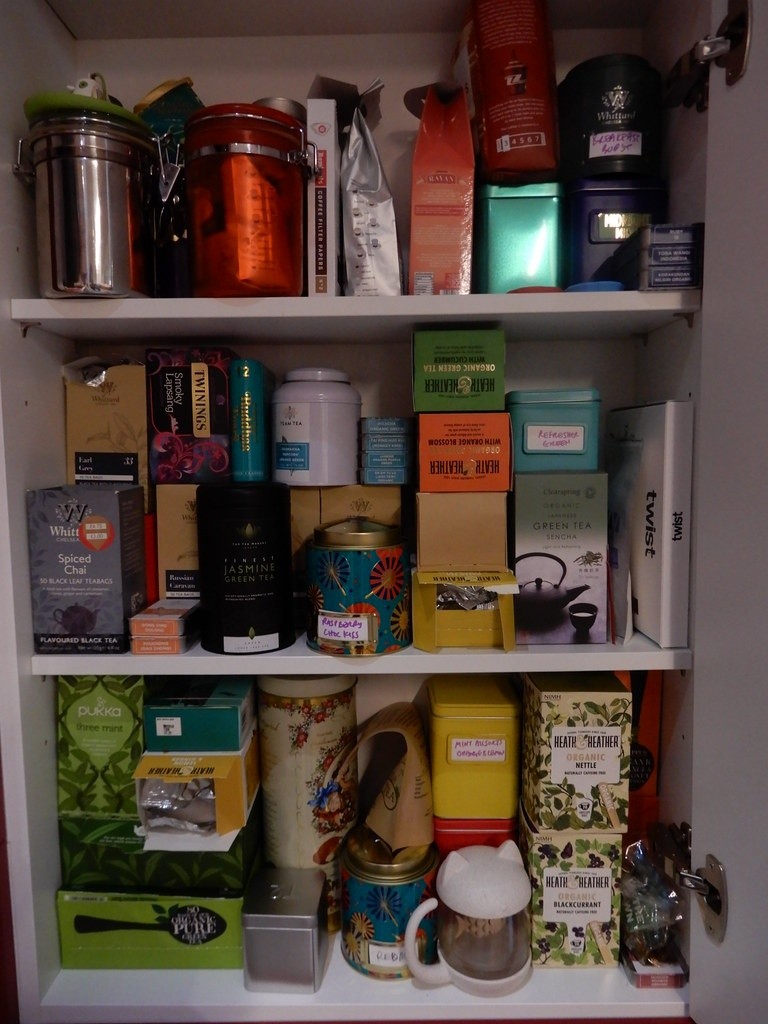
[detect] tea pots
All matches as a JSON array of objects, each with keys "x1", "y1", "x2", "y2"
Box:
[{"x1": 511, "y1": 552, "x2": 592, "y2": 633}]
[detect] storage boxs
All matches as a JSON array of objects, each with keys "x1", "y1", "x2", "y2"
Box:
[
  {"x1": 615, "y1": 670, "x2": 663, "y2": 797},
  {"x1": 142, "y1": 677, "x2": 253, "y2": 752},
  {"x1": 409, "y1": 328, "x2": 507, "y2": 412},
  {"x1": 62, "y1": 351, "x2": 155, "y2": 515},
  {"x1": 57, "y1": 791, "x2": 263, "y2": 898},
  {"x1": 55, "y1": 675, "x2": 147, "y2": 819},
  {"x1": 320, "y1": 487, "x2": 402, "y2": 526},
  {"x1": 513, "y1": 470, "x2": 607, "y2": 645},
  {"x1": 418, "y1": 412, "x2": 515, "y2": 493},
  {"x1": 239, "y1": 866, "x2": 329, "y2": 994},
  {"x1": 414, "y1": 491, "x2": 509, "y2": 568},
  {"x1": 156, "y1": 484, "x2": 200, "y2": 601},
  {"x1": 56, "y1": 891, "x2": 245, "y2": 970},
  {"x1": 411, "y1": 567, "x2": 520, "y2": 654},
  {"x1": 289, "y1": 486, "x2": 320, "y2": 597},
  {"x1": 131, "y1": 735, "x2": 264, "y2": 850},
  {"x1": 605, "y1": 399, "x2": 695, "y2": 649},
  {"x1": 305, "y1": 97, "x2": 701, "y2": 297},
  {"x1": 143, "y1": 345, "x2": 241, "y2": 487},
  {"x1": 24, "y1": 483, "x2": 146, "y2": 656},
  {"x1": 423, "y1": 671, "x2": 633, "y2": 970},
  {"x1": 505, "y1": 386, "x2": 602, "y2": 475}
]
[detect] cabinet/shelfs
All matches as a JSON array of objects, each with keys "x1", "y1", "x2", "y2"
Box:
[{"x1": 0, "y1": 0, "x2": 768, "y2": 1024}]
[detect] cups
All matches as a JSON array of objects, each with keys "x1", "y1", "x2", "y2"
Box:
[
  {"x1": 402, "y1": 838, "x2": 531, "y2": 997},
  {"x1": 569, "y1": 603, "x2": 599, "y2": 631}
]
